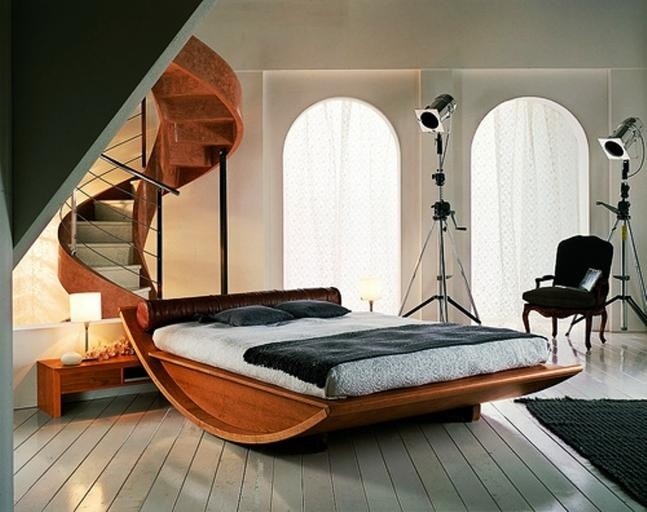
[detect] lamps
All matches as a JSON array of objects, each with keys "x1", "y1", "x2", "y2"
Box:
[
  {"x1": 68, "y1": 293, "x2": 101, "y2": 360},
  {"x1": 359, "y1": 277, "x2": 383, "y2": 311}
]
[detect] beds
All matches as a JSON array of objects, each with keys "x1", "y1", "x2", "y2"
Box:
[{"x1": 117, "y1": 287, "x2": 584, "y2": 445}]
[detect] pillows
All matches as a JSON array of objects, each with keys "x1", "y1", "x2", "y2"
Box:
[
  {"x1": 273, "y1": 300, "x2": 351, "y2": 317},
  {"x1": 212, "y1": 305, "x2": 294, "y2": 326}
]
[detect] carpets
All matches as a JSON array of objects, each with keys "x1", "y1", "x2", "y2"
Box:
[{"x1": 514, "y1": 396, "x2": 647, "y2": 509}]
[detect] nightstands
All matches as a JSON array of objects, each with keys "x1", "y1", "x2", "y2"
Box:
[{"x1": 37, "y1": 350, "x2": 153, "y2": 418}]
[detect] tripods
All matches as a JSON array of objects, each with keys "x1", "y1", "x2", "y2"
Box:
[
  {"x1": 395, "y1": 152, "x2": 485, "y2": 338},
  {"x1": 567, "y1": 177, "x2": 647, "y2": 358}
]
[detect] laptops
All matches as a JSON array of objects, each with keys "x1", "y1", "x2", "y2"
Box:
[{"x1": 554, "y1": 267, "x2": 603, "y2": 294}]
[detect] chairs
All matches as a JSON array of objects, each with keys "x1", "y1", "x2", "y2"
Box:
[{"x1": 522, "y1": 235, "x2": 614, "y2": 349}]
